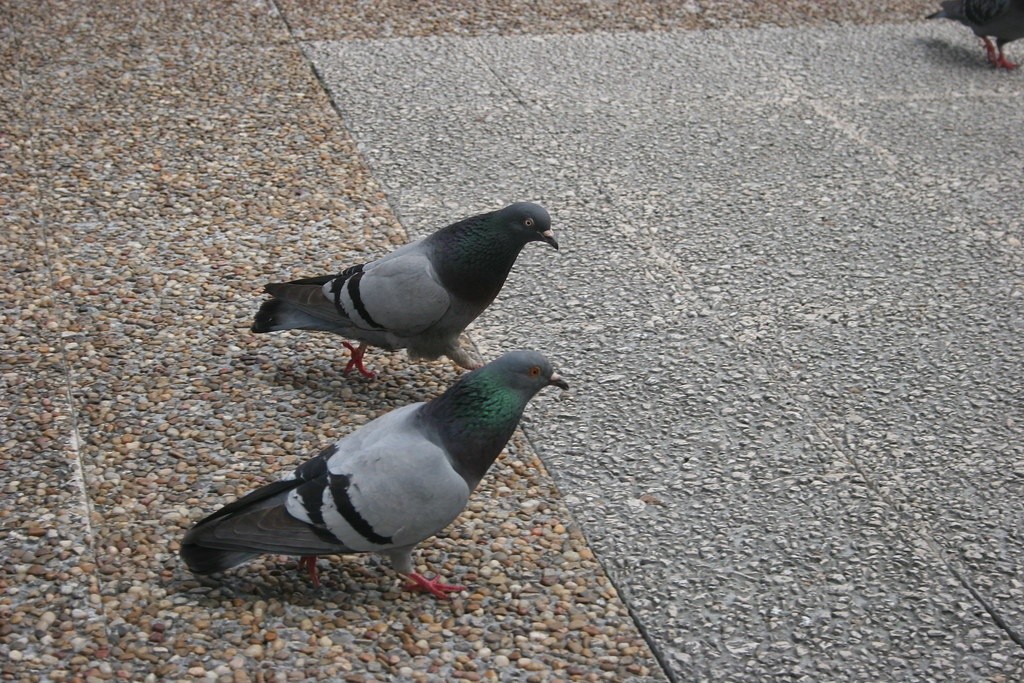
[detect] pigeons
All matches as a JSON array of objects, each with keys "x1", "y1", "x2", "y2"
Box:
[
  {"x1": 178, "y1": 350, "x2": 569, "y2": 601},
  {"x1": 925, "y1": 1, "x2": 1024, "y2": 72},
  {"x1": 250, "y1": 202, "x2": 558, "y2": 374}
]
